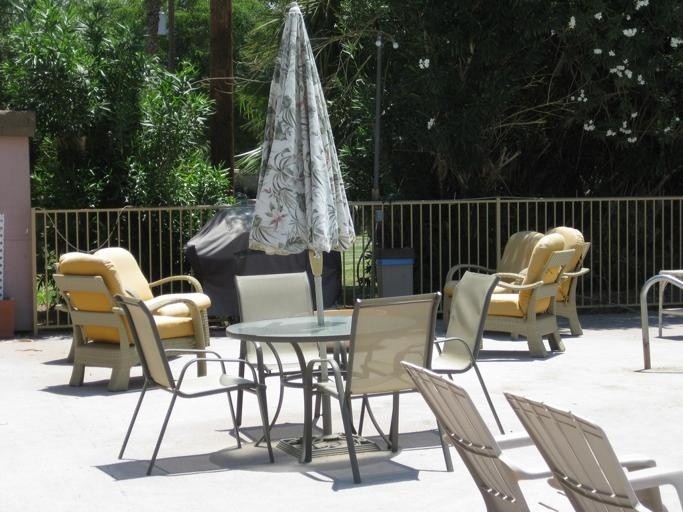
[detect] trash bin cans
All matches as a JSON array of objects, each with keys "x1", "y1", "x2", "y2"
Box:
[{"x1": 375, "y1": 247, "x2": 415, "y2": 296}]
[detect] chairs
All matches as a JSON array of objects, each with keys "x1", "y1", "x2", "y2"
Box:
[
  {"x1": 443, "y1": 225, "x2": 591, "y2": 357},
  {"x1": 222, "y1": 273, "x2": 504, "y2": 464},
  {"x1": 113, "y1": 295, "x2": 276, "y2": 476},
  {"x1": 52, "y1": 245, "x2": 211, "y2": 390},
  {"x1": 299, "y1": 291, "x2": 454, "y2": 483},
  {"x1": 400, "y1": 360, "x2": 663, "y2": 510},
  {"x1": 504, "y1": 390, "x2": 682, "y2": 511}
]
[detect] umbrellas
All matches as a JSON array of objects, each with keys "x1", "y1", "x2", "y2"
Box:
[{"x1": 246, "y1": 1, "x2": 359, "y2": 437}]
